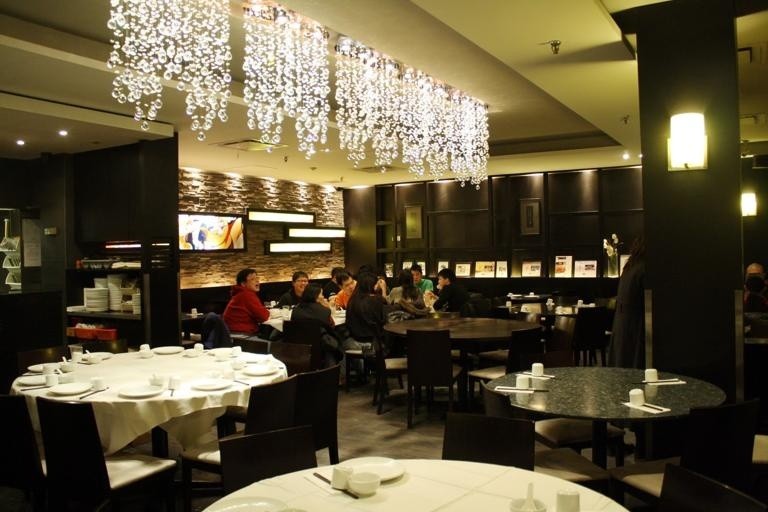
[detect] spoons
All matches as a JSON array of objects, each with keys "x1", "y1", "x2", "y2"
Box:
[{"x1": 522, "y1": 482, "x2": 536, "y2": 512}]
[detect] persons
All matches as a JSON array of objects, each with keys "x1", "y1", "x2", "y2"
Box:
[
  {"x1": 745, "y1": 262, "x2": 764, "y2": 275},
  {"x1": 185, "y1": 219, "x2": 206, "y2": 250},
  {"x1": 223, "y1": 264, "x2": 464, "y2": 386},
  {"x1": 743, "y1": 273, "x2": 768, "y2": 313},
  {"x1": 606, "y1": 229, "x2": 646, "y2": 456}
]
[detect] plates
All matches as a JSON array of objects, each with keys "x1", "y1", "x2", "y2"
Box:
[
  {"x1": 200, "y1": 497, "x2": 291, "y2": 512},
  {"x1": 325, "y1": 293, "x2": 348, "y2": 318},
  {"x1": 65, "y1": 274, "x2": 141, "y2": 313},
  {"x1": 266, "y1": 301, "x2": 296, "y2": 318},
  {"x1": 17, "y1": 343, "x2": 283, "y2": 400},
  {"x1": 335, "y1": 457, "x2": 407, "y2": 483}
]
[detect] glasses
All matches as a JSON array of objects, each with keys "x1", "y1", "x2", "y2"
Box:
[
  {"x1": 248, "y1": 277, "x2": 260, "y2": 281},
  {"x1": 296, "y1": 279, "x2": 307, "y2": 283}
]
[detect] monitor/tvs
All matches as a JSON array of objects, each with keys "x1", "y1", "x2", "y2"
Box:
[{"x1": 178, "y1": 211, "x2": 249, "y2": 254}]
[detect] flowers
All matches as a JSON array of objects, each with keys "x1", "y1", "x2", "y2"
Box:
[{"x1": 602, "y1": 233, "x2": 619, "y2": 257}]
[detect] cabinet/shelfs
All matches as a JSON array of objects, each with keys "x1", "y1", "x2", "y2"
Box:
[{"x1": 62, "y1": 239, "x2": 180, "y2": 357}]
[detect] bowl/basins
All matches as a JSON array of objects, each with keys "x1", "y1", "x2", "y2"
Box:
[
  {"x1": 508, "y1": 496, "x2": 548, "y2": 511},
  {"x1": 348, "y1": 474, "x2": 380, "y2": 497}
]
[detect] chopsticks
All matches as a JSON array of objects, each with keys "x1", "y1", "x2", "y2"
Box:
[
  {"x1": 620, "y1": 398, "x2": 662, "y2": 412},
  {"x1": 516, "y1": 372, "x2": 555, "y2": 379},
  {"x1": 631, "y1": 380, "x2": 679, "y2": 385},
  {"x1": 313, "y1": 471, "x2": 358, "y2": 501},
  {"x1": 495, "y1": 388, "x2": 550, "y2": 393}
]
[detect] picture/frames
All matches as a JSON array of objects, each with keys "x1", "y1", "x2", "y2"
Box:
[
  {"x1": 520, "y1": 197, "x2": 541, "y2": 236},
  {"x1": 403, "y1": 206, "x2": 424, "y2": 240}
]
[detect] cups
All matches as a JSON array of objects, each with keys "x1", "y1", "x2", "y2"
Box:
[
  {"x1": 644, "y1": 368, "x2": 657, "y2": 381},
  {"x1": 628, "y1": 389, "x2": 644, "y2": 407},
  {"x1": 503, "y1": 292, "x2": 597, "y2": 315},
  {"x1": 531, "y1": 363, "x2": 543, "y2": 377},
  {"x1": 555, "y1": 490, "x2": 579, "y2": 512},
  {"x1": 515, "y1": 394, "x2": 548, "y2": 410},
  {"x1": 515, "y1": 375, "x2": 529, "y2": 390},
  {"x1": 330, "y1": 465, "x2": 351, "y2": 491}
]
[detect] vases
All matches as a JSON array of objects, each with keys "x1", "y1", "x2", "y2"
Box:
[{"x1": 607, "y1": 255, "x2": 619, "y2": 278}]
